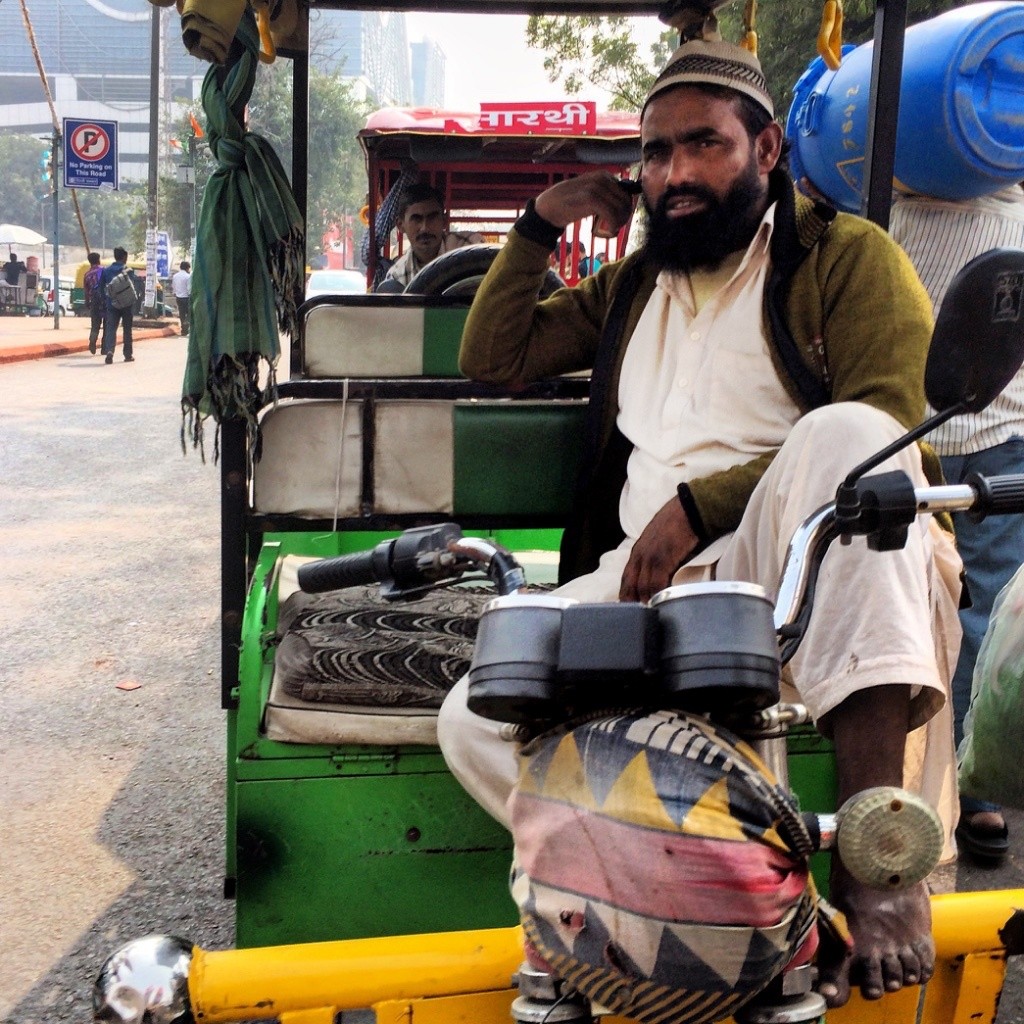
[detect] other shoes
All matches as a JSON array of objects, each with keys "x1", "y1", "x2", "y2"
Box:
[
  {"x1": 89, "y1": 343, "x2": 96, "y2": 355},
  {"x1": 125, "y1": 356, "x2": 134, "y2": 361},
  {"x1": 105, "y1": 353, "x2": 113, "y2": 363}
]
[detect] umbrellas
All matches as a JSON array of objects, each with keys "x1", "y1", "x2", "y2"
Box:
[{"x1": 0, "y1": 223, "x2": 48, "y2": 253}]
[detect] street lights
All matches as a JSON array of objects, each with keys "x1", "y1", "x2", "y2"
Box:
[{"x1": 42, "y1": 200, "x2": 65, "y2": 268}]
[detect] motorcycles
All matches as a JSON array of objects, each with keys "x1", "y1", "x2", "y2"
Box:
[{"x1": 93, "y1": 0, "x2": 1024, "y2": 1023}]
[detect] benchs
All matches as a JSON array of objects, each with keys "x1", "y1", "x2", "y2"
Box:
[
  {"x1": 235, "y1": 380, "x2": 591, "y2": 750},
  {"x1": 287, "y1": 292, "x2": 474, "y2": 377}
]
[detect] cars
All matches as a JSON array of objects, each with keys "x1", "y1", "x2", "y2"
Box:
[{"x1": 306, "y1": 271, "x2": 367, "y2": 299}]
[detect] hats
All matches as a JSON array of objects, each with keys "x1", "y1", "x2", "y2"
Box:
[{"x1": 639, "y1": 40, "x2": 774, "y2": 121}]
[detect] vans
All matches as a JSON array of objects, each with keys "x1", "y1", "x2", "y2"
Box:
[
  {"x1": 38, "y1": 277, "x2": 75, "y2": 317},
  {"x1": 71, "y1": 261, "x2": 163, "y2": 318}
]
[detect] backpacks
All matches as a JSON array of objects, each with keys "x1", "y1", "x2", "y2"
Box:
[{"x1": 104, "y1": 268, "x2": 138, "y2": 308}]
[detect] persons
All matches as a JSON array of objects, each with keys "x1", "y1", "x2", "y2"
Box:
[
  {"x1": 880, "y1": 182, "x2": 1024, "y2": 867},
  {"x1": 549, "y1": 240, "x2": 610, "y2": 281},
  {"x1": 385, "y1": 183, "x2": 487, "y2": 288},
  {"x1": 0, "y1": 253, "x2": 27, "y2": 303},
  {"x1": 437, "y1": 39, "x2": 948, "y2": 1009},
  {"x1": 98, "y1": 247, "x2": 136, "y2": 364},
  {"x1": 84, "y1": 253, "x2": 108, "y2": 356},
  {"x1": 172, "y1": 262, "x2": 191, "y2": 336}
]
